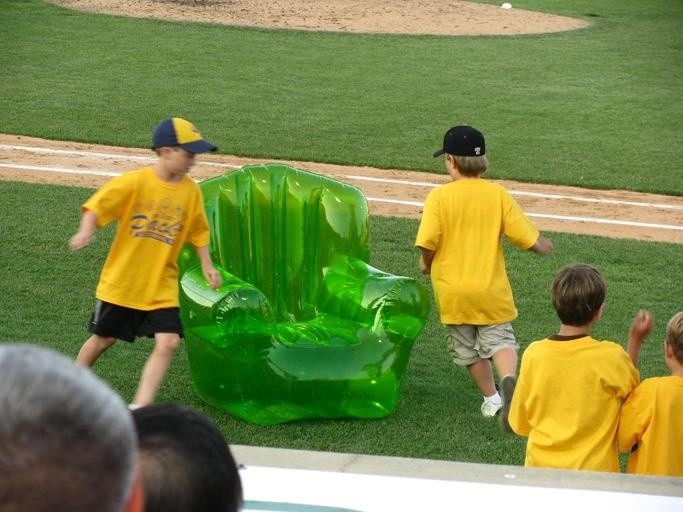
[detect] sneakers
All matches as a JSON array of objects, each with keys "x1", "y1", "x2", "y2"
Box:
[
  {"x1": 495, "y1": 374, "x2": 517, "y2": 432},
  {"x1": 479, "y1": 399, "x2": 503, "y2": 417}
]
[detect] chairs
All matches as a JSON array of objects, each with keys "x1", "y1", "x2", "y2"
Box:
[{"x1": 177, "y1": 162, "x2": 431, "y2": 428}]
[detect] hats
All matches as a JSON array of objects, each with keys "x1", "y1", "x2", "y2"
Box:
[
  {"x1": 150, "y1": 117, "x2": 217, "y2": 154},
  {"x1": 432, "y1": 126, "x2": 485, "y2": 157}
]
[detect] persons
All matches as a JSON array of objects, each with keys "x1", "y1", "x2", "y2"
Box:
[
  {"x1": 124, "y1": 404, "x2": 242, "y2": 511},
  {"x1": 616, "y1": 312, "x2": 683, "y2": 478},
  {"x1": 1, "y1": 343, "x2": 138, "y2": 511},
  {"x1": 67, "y1": 117, "x2": 223, "y2": 410},
  {"x1": 507, "y1": 263, "x2": 653, "y2": 473},
  {"x1": 415, "y1": 126, "x2": 554, "y2": 433}
]
